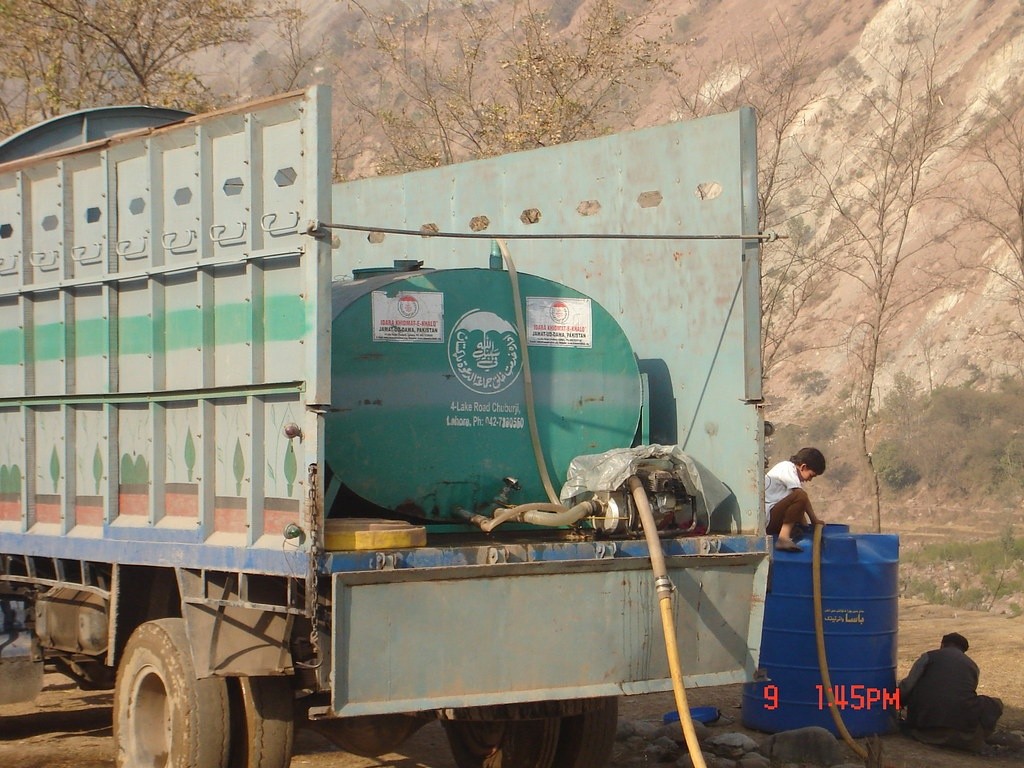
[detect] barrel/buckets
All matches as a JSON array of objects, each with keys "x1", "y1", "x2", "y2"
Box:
[{"x1": 742, "y1": 524, "x2": 899, "y2": 738}]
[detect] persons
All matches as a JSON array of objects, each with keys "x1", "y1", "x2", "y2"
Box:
[
  {"x1": 898, "y1": 632, "x2": 1004, "y2": 735},
  {"x1": 765, "y1": 447, "x2": 826, "y2": 552}
]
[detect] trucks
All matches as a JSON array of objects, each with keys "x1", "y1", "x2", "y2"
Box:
[{"x1": 0, "y1": 81, "x2": 778, "y2": 768}]
